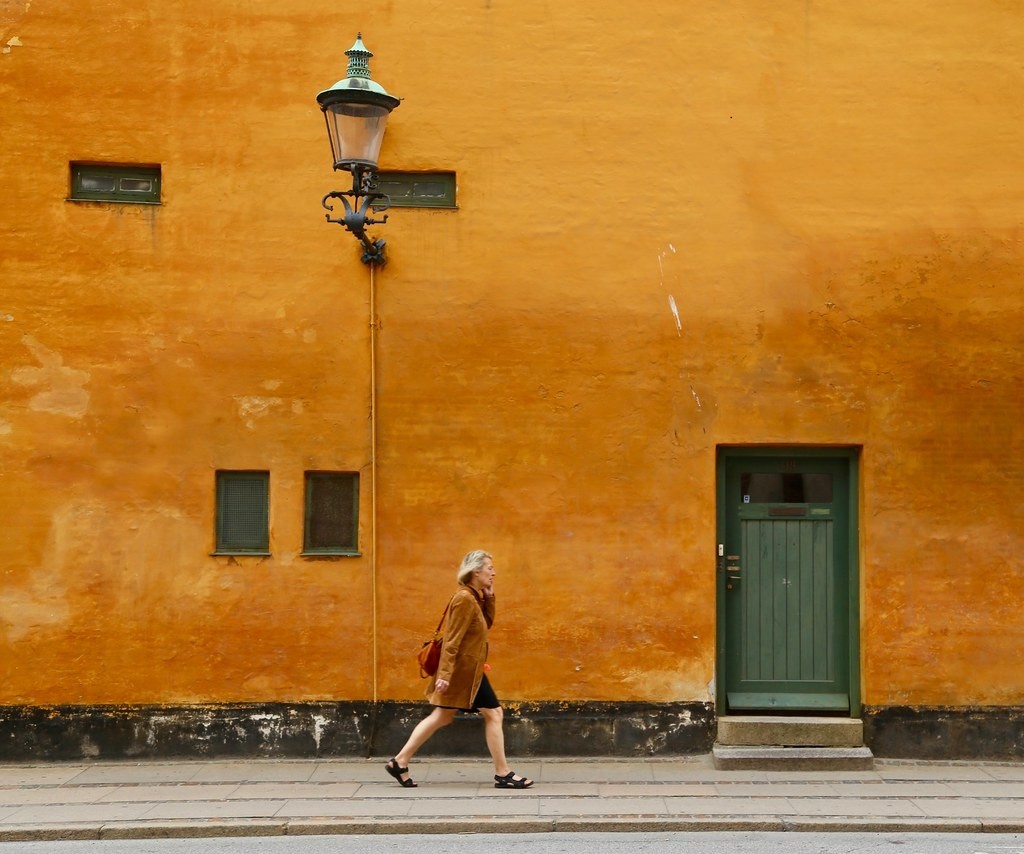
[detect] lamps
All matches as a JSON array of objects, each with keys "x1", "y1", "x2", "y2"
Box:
[{"x1": 316, "y1": 34, "x2": 406, "y2": 268}]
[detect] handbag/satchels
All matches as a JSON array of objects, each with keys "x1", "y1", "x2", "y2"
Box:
[{"x1": 416, "y1": 639, "x2": 442, "y2": 679}]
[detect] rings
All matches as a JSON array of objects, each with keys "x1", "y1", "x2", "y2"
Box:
[{"x1": 438, "y1": 684, "x2": 442, "y2": 687}]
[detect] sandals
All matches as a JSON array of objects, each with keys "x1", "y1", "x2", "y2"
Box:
[
  {"x1": 385, "y1": 757, "x2": 418, "y2": 788},
  {"x1": 493, "y1": 770, "x2": 534, "y2": 789}
]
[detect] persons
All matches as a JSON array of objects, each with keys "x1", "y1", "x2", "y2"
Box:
[{"x1": 385, "y1": 550, "x2": 534, "y2": 788}]
